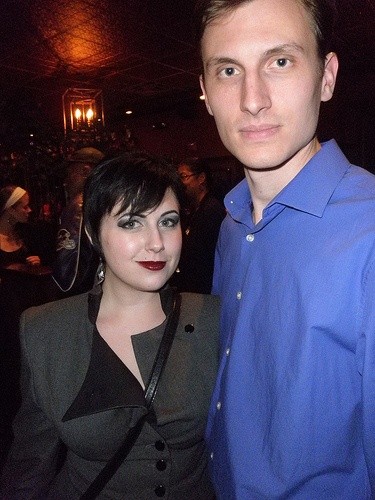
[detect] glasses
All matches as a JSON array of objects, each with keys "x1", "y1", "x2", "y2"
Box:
[{"x1": 179, "y1": 171, "x2": 203, "y2": 180}]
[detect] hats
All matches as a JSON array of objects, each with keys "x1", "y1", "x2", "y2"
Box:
[{"x1": 50, "y1": 147, "x2": 105, "y2": 174}]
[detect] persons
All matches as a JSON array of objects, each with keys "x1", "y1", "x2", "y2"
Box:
[
  {"x1": 2, "y1": 150, "x2": 221, "y2": 500},
  {"x1": 195, "y1": 0, "x2": 375, "y2": 500},
  {"x1": 0, "y1": 132, "x2": 226, "y2": 290}
]
